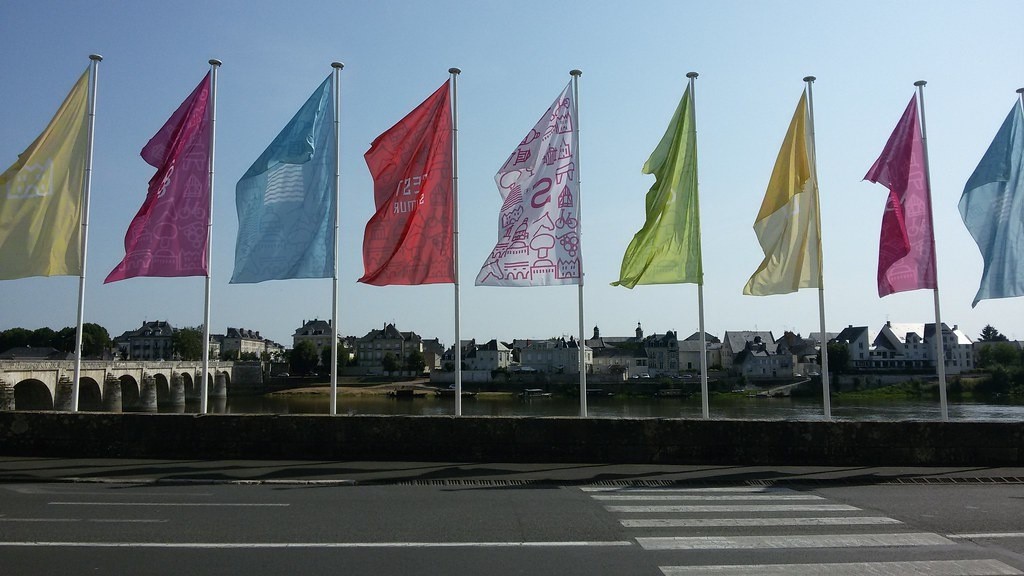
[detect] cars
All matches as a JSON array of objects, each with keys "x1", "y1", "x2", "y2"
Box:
[
  {"x1": 628, "y1": 375, "x2": 639, "y2": 379},
  {"x1": 449, "y1": 384, "x2": 456, "y2": 389},
  {"x1": 808, "y1": 371, "x2": 820, "y2": 376},
  {"x1": 678, "y1": 374, "x2": 693, "y2": 379},
  {"x1": 792, "y1": 372, "x2": 801, "y2": 377},
  {"x1": 639, "y1": 372, "x2": 651, "y2": 379}
]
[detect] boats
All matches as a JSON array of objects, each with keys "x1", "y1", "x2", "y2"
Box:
[
  {"x1": 657, "y1": 389, "x2": 689, "y2": 398},
  {"x1": 584, "y1": 388, "x2": 616, "y2": 400},
  {"x1": 518, "y1": 388, "x2": 553, "y2": 399}
]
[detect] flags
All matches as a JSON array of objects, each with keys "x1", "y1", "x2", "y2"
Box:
[
  {"x1": 862, "y1": 81, "x2": 948, "y2": 299},
  {"x1": 102, "y1": 59, "x2": 223, "y2": 284},
  {"x1": 611, "y1": 73, "x2": 709, "y2": 289},
  {"x1": 0, "y1": 54, "x2": 102, "y2": 280},
  {"x1": 474, "y1": 70, "x2": 586, "y2": 287},
  {"x1": 229, "y1": 63, "x2": 344, "y2": 284},
  {"x1": 357, "y1": 68, "x2": 462, "y2": 286},
  {"x1": 958, "y1": 89, "x2": 1024, "y2": 308},
  {"x1": 743, "y1": 76, "x2": 831, "y2": 296}
]
